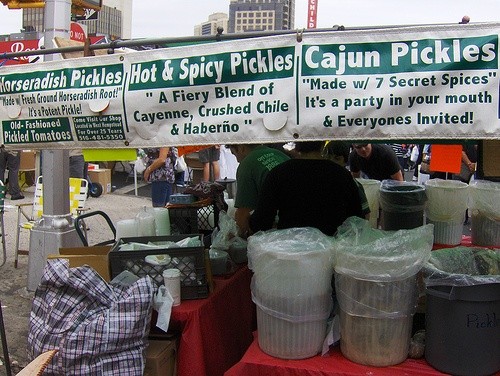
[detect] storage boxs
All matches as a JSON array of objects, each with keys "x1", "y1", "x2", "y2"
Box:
[
  {"x1": 170, "y1": 192, "x2": 196, "y2": 204},
  {"x1": 20, "y1": 152, "x2": 35, "y2": 169},
  {"x1": 187, "y1": 157, "x2": 203, "y2": 168},
  {"x1": 88, "y1": 165, "x2": 112, "y2": 195},
  {"x1": 48, "y1": 245, "x2": 113, "y2": 284},
  {"x1": 145, "y1": 338, "x2": 178, "y2": 376},
  {"x1": 169, "y1": 202, "x2": 220, "y2": 248},
  {"x1": 110, "y1": 234, "x2": 208, "y2": 300}
]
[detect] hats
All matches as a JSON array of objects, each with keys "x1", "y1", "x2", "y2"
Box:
[
  {"x1": 324, "y1": 141, "x2": 350, "y2": 155},
  {"x1": 352, "y1": 142, "x2": 369, "y2": 148}
]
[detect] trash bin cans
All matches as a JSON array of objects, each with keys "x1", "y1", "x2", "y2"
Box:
[
  {"x1": 420, "y1": 245, "x2": 499, "y2": 375},
  {"x1": 426, "y1": 179, "x2": 468, "y2": 246},
  {"x1": 470, "y1": 180, "x2": 499, "y2": 246},
  {"x1": 357, "y1": 177, "x2": 379, "y2": 231},
  {"x1": 379, "y1": 183, "x2": 427, "y2": 232},
  {"x1": 251, "y1": 242, "x2": 333, "y2": 359},
  {"x1": 335, "y1": 264, "x2": 421, "y2": 367}
]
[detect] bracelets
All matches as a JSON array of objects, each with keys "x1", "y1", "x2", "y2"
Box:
[
  {"x1": 148, "y1": 167, "x2": 152, "y2": 171},
  {"x1": 468, "y1": 162, "x2": 472, "y2": 166}
]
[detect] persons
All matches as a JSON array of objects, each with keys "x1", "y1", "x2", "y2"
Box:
[
  {"x1": 224, "y1": 143, "x2": 291, "y2": 239},
  {"x1": 250, "y1": 141, "x2": 364, "y2": 237},
  {"x1": 0, "y1": 141, "x2": 476, "y2": 219}
]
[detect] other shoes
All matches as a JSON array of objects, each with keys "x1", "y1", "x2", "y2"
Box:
[{"x1": 11, "y1": 193, "x2": 25, "y2": 200}]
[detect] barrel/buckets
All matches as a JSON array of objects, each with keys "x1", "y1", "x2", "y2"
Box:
[{"x1": 216, "y1": 177, "x2": 235, "y2": 199}]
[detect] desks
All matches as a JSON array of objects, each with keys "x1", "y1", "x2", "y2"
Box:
[
  {"x1": 18, "y1": 169, "x2": 36, "y2": 187},
  {"x1": 187, "y1": 167, "x2": 204, "y2": 186},
  {"x1": 151, "y1": 262, "x2": 258, "y2": 376},
  {"x1": 225, "y1": 244, "x2": 500, "y2": 376}
]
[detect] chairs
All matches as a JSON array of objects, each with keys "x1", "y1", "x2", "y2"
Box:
[{"x1": 14, "y1": 176, "x2": 90, "y2": 268}]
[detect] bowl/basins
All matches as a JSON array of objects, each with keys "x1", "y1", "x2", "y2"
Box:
[{"x1": 113, "y1": 207, "x2": 171, "y2": 241}]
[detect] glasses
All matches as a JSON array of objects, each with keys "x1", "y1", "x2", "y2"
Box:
[{"x1": 355, "y1": 144, "x2": 368, "y2": 149}]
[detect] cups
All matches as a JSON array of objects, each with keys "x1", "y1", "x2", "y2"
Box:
[{"x1": 163, "y1": 269, "x2": 181, "y2": 307}]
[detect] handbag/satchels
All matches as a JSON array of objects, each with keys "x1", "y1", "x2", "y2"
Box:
[
  {"x1": 173, "y1": 155, "x2": 187, "y2": 173},
  {"x1": 27, "y1": 259, "x2": 153, "y2": 376}
]
